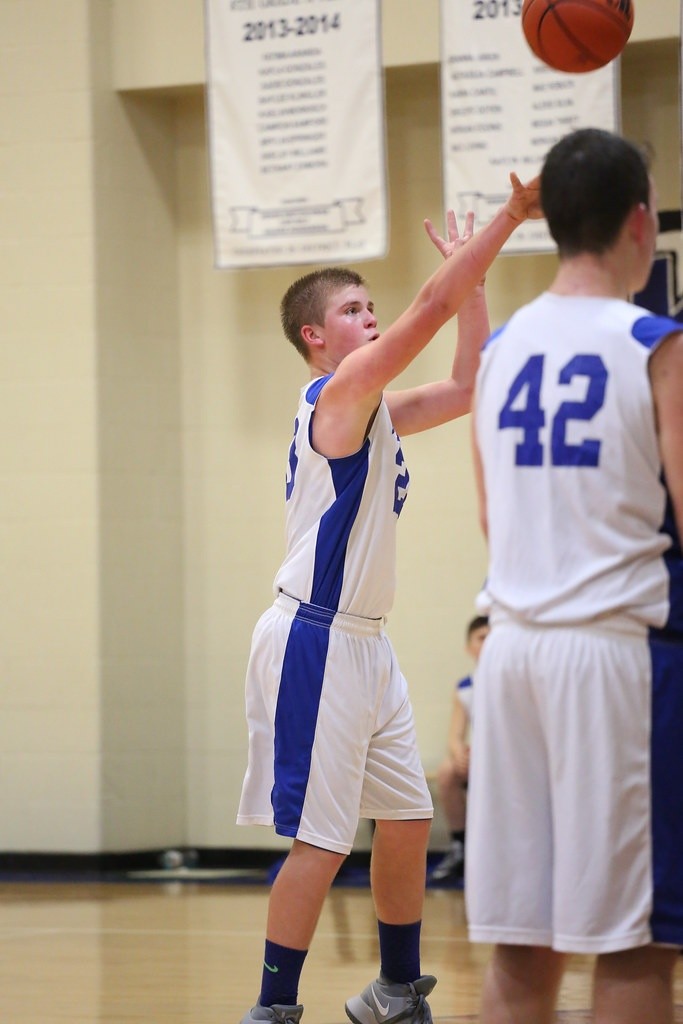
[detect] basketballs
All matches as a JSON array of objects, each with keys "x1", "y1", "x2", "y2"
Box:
[{"x1": 522, "y1": 0, "x2": 635, "y2": 73}]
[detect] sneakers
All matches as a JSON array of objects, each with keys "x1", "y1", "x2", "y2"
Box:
[
  {"x1": 344, "y1": 975, "x2": 438, "y2": 1024},
  {"x1": 432, "y1": 841, "x2": 465, "y2": 880},
  {"x1": 238, "y1": 994, "x2": 304, "y2": 1024}
]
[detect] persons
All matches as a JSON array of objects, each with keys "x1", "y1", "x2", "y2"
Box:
[
  {"x1": 244, "y1": 171, "x2": 552, "y2": 1024},
  {"x1": 429, "y1": 614, "x2": 497, "y2": 882},
  {"x1": 461, "y1": 127, "x2": 683, "y2": 1024}
]
